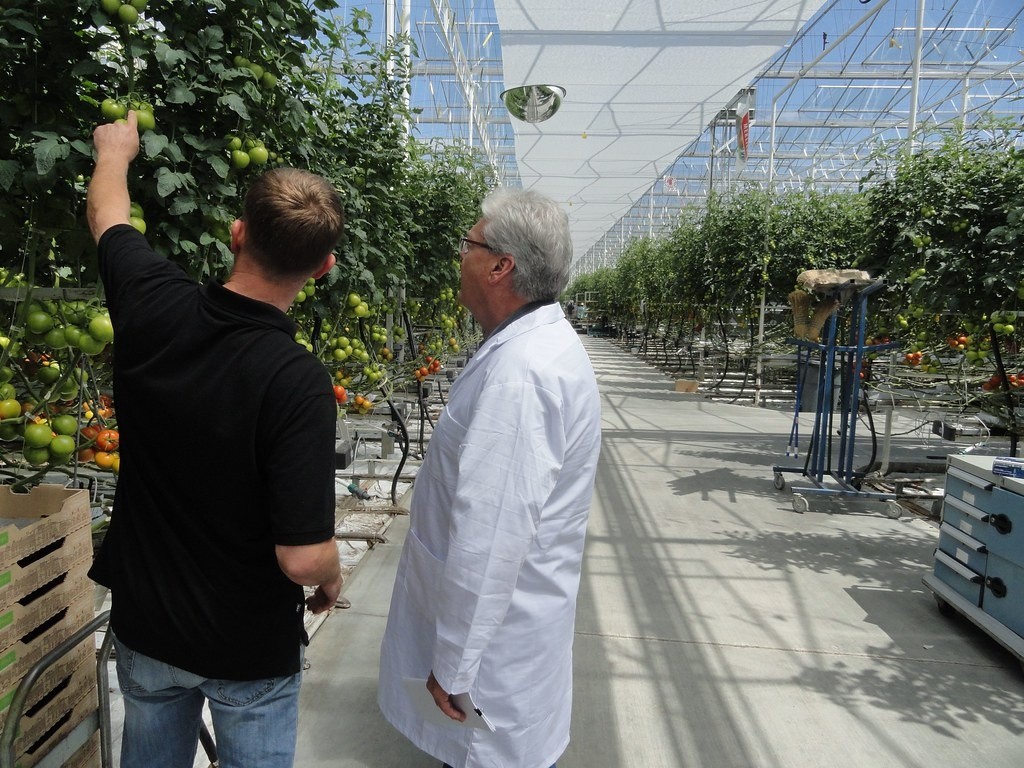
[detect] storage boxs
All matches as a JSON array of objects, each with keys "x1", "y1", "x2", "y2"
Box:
[{"x1": 0, "y1": 482, "x2": 100, "y2": 768}]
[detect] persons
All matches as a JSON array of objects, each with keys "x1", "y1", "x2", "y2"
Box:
[
  {"x1": 576, "y1": 302, "x2": 584, "y2": 320},
  {"x1": 378, "y1": 190, "x2": 601, "y2": 768},
  {"x1": 567, "y1": 303, "x2": 574, "y2": 320},
  {"x1": 86, "y1": 109, "x2": 344, "y2": 768}
]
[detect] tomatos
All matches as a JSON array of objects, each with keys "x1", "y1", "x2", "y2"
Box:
[
  {"x1": 0, "y1": 0, "x2": 473, "y2": 473},
  {"x1": 854, "y1": 191, "x2": 1024, "y2": 392}
]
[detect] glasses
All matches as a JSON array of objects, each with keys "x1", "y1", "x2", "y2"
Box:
[{"x1": 457, "y1": 235, "x2": 507, "y2": 257}]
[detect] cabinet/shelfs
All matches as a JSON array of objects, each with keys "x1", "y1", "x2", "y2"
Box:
[{"x1": 922, "y1": 454, "x2": 1024, "y2": 662}]
